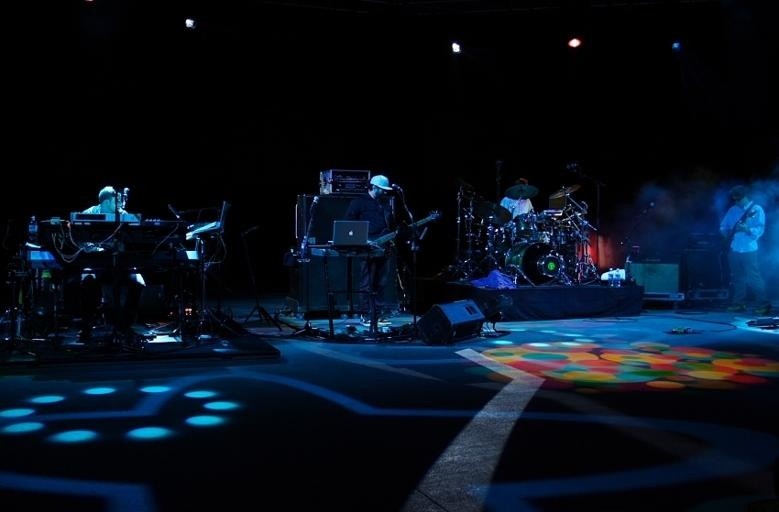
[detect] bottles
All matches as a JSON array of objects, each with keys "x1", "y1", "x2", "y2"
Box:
[
  {"x1": 607, "y1": 267, "x2": 621, "y2": 289},
  {"x1": 27, "y1": 215, "x2": 38, "y2": 242},
  {"x1": 319, "y1": 170, "x2": 331, "y2": 195}
]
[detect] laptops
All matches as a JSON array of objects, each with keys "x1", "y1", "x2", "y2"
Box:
[{"x1": 328, "y1": 220, "x2": 370, "y2": 245}]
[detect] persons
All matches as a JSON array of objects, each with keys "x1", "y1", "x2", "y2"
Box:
[
  {"x1": 500, "y1": 179, "x2": 537, "y2": 284},
  {"x1": 720, "y1": 186, "x2": 771, "y2": 315},
  {"x1": 81, "y1": 185, "x2": 151, "y2": 359},
  {"x1": 343, "y1": 174, "x2": 395, "y2": 325}
]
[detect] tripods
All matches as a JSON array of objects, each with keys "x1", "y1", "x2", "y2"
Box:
[
  {"x1": 433, "y1": 188, "x2": 610, "y2": 287},
  {"x1": 243, "y1": 288, "x2": 281, "y2": 333},
  {"x1": 172, "y1": 260, "x2": 236, "y2": 340},
  {"x1": 290, "y1": 212, "x2": 325, "y2": 344}
]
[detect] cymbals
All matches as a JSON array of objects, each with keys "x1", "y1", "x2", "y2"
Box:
[
  {"x1": 477, "y1": 203, "x2": 511, "y2": 225},
  {"x1": 506, "y1": 186, "x2": 538, "y2": 199},
  {"x1": 549, "y1": 185, "x2": 579, "y2": 199}
]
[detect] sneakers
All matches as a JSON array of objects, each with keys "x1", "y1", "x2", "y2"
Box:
[
  {"x1": 360, "y1": 314, "x2": 372, "y2": 327},
  {"x1": 378, "y1": 316, "x2": 392, "y2": 325}
]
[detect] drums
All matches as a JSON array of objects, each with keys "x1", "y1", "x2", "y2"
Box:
[
  {"x1": 514, "y1": 213, "x2": 553, "y2": 242},
  {"x1": 505, "y1": 243, "x2": 556, "y2": 286}
]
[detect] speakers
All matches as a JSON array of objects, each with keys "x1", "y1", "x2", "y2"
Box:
[{"x1": 420, "y1": 299, "x2": 489, "y2": 340}]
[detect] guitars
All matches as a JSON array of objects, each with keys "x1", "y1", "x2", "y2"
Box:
[
  {"x1": 719, "y1": 210, "x2": 757, "y2": 255},
  {"x1": 373, "y1": 210, "x2": 440, "y2": 256}
]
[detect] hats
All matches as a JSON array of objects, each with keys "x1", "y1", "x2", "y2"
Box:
[{"x1": 370, "y1": 176, "x2": 393, "y2": 190}]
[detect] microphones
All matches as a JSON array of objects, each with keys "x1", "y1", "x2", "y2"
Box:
[
  {"x1": 310, "y1": 193, "x2": 322, "y2": 210},
  {"x1": 121, "y1": 187, "x2": 130, "y2": 208},
  {"x1": 391, "y1": 183, "x2": 401, "y2": 190}
]
